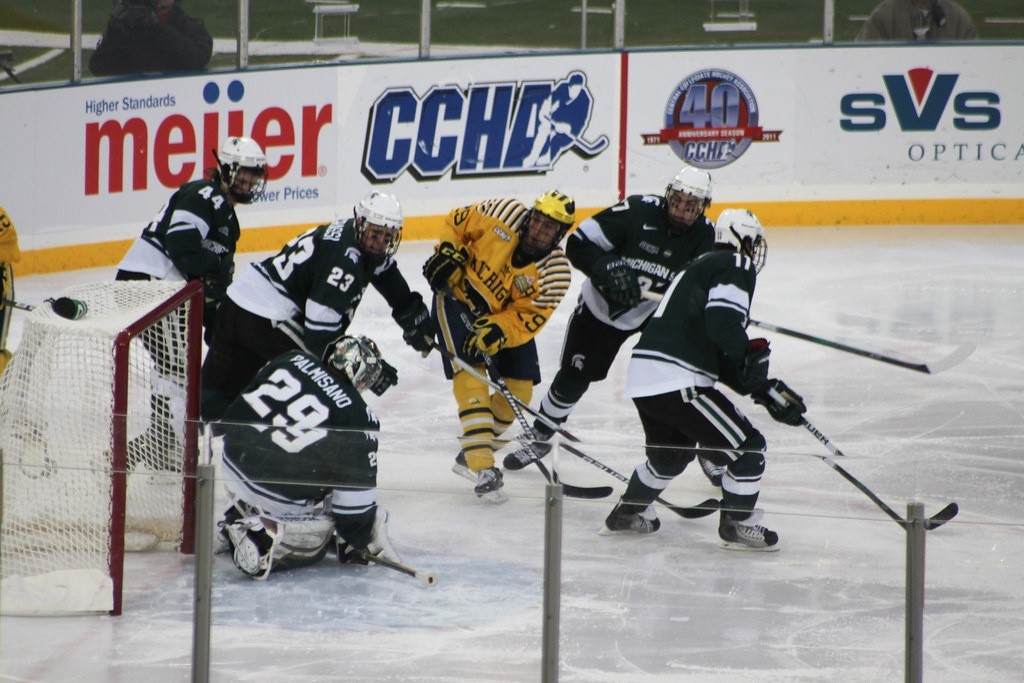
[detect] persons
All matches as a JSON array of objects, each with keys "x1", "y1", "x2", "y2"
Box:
[
  {"x1": 854, "y1": 0, "x2": 979, "y2": 41},
  {"x1": 606, "y1": 207, "x2": 807, "y2": 549},
  {"x1": 113, "y1": 135, "x2": 268, "y2": 470},
  {"x1": 502, "y1": 164, "x2": 727, "y2": 486},
  {"x1": 89, "y1": 187, "x2": 435, "y2": 486},
  {"x1": 0, "y1": 209, "x2": 22, "y2": 371},
  {"x1": 212, "y1": 334, "x2": 398, "y2": 577},
  {"x1": 422, "y1": 190, "x2": 576, "y2": 498},
  {"x1": 87, "y1": 0, "x2": 214, "y2": 76}
]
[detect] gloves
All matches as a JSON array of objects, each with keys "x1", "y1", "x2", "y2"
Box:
[
  {"x1": 462, "y1": 316, "x2": 505, "y2": 358},
  {"x1": 368, "y1": 358, "x2": 397, "y2": 396},
  {"x1": 423, "y1": 246, "x2": 468, "y2": 298},
  {"x1": 736, "y1": 338, "x2": 771, "y2": 394},
  {"x1": 753, "y1": 378, "x2": 807, "y2": 426},
  {"x1": 391, "y1": 292, "x2": 435, "y2": 358},
  {"x1": 601, "y1": 260, "x2": 640, "y2": 306}
]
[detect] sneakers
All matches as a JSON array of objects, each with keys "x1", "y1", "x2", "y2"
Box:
[
  {"x1": 504, "y1": 427, "x2": 552, "y2": 471},
  {"x1": 599, "y1": 497, "x2": 661, "y2": 537},
  {"x1": 475, "y1": 468, "x2": 510, "y2": 503},
  {"x1": 697, "y1": 443, "x2": 726, "y2": 487},
  {"x1": 717, "y1": 519, "x2": 781, "y2": 552},
  {"x1": 451, "y1": 450, "x2": 479, "y2": 481}
]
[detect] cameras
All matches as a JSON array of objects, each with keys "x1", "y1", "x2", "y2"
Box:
[{"x1": 121, "y1": 0, "x2": 154, "y2": 27}]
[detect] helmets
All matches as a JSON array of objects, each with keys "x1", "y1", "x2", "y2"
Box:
[
  {"x1": 665, "y1": 166, "x2": 713, "y2": 234},
  {"x1": 328, "y1": 336, "x2": 376, "y2": 389},
  {"x1": 714, "y1": 209, "x2": 768, "y2": 274},
  {"x1": 352, "y1": 191, "x2": 403, "y2": 262},
  {"x1": 521, "y1": 189, "x2": 575, "y2": 259},
  {"x1": 212, "y1": 136, "x2": 268, "y2": 204}
]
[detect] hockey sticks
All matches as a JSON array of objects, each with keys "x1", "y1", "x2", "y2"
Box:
[
  {"x1": 422, "y1": 332, "x2": 582, "y2": 442},
  {"x1": 4, "y1": 299, "x2": 35, "y2": 311},
  {"x1": 460, "y1": 312, "x2": 613, "y2": 499},
  {"x1": 362, "y1": 551, "x2": 435, "y2": 583},
  {"x1": 758, "y1": 376, "x2": 959, "y2": 531},
  {"x1": 639, "y1": 289, "x2": 976, "y2": 373},
  {"x1": 559, "y1": 441, "x2": 721, "y2": 519}
]
